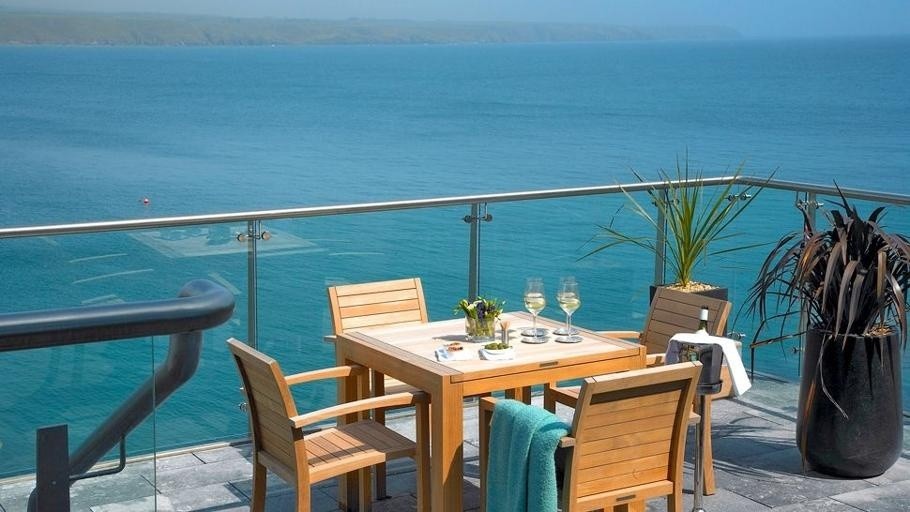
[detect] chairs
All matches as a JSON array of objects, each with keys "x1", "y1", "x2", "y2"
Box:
[{"x1": 226, "y1": 277, "x2": 732, "y2": 512}]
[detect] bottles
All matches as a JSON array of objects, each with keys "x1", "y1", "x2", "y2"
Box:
[{"x1": 696, "y1": 304, "x2": 710, "y2": 334}]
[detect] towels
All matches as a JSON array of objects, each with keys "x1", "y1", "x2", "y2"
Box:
[{"x1": 664, "y1": 332, "x2": 752, "y2": 397}]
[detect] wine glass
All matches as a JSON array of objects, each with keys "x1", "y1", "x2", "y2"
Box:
[
  {"x1": 521, "y1": 282, "x2": 549, "y2": 344},
  {"x1": 555, "y1": 282, "x2": 583, "y2": 343},
  {"x1": 521, "y1": 274, "x2": 548, "y2": 336},
  {"x1": 552, "y1": 272, "x2": 580, "y2": 335}
]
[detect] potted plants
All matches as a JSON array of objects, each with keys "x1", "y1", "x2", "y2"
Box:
[
  {"x1": 731, "y1": 180, "x2": 910, "y2": 479},
  {"x1": 575, "y1": 147, "x2": 781, "y2": 337}
]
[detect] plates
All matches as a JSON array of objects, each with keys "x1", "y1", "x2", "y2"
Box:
[{"x1": 481, "y1": 345, "x2": 513, "y2": 355}]
